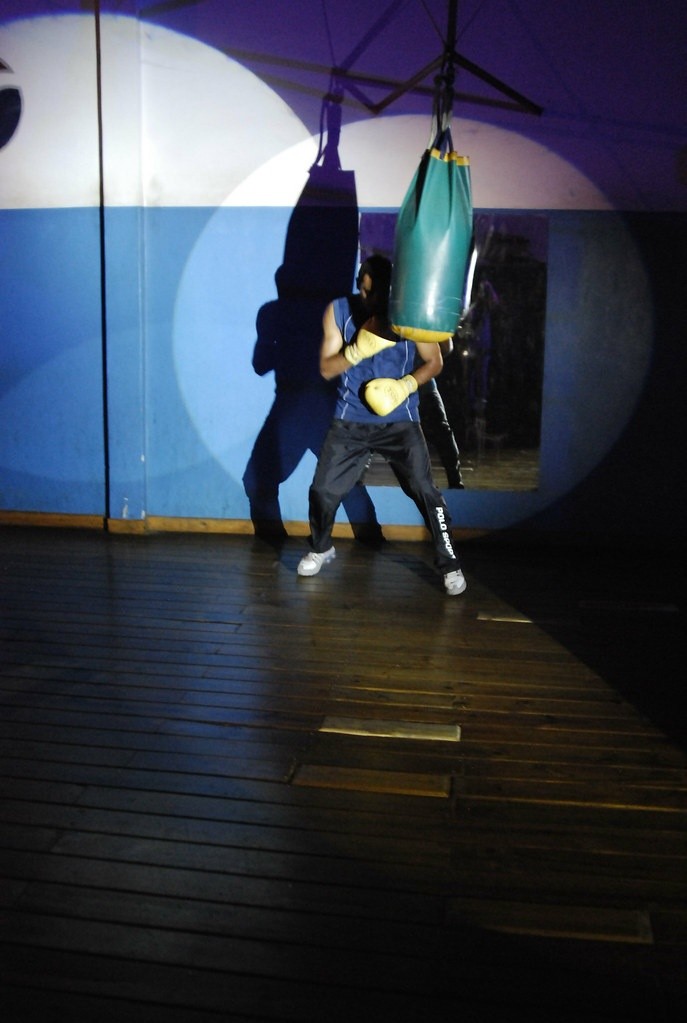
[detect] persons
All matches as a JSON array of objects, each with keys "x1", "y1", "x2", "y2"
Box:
[
  {"x1": 298, "y1": 255, "x2": 466, "y2": 595},
  {"x1": 360, "y1": 329, "x2": 465, "y2": 489}
]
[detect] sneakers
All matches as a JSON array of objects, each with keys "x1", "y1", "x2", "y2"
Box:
[
  {"x1": 297, "y1": 546, "x2": 336, "y2": 576},
  {"x1": 443, "y1": 568, "x2": 467, "y2": 596}
]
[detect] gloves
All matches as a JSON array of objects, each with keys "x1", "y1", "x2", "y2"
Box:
[
  {"x1": 365, "y1": 374, "x2": 418, "y2": 416},
  {"x1": 344, "y1": 315, "x2": 397, "y2": 366}
]
